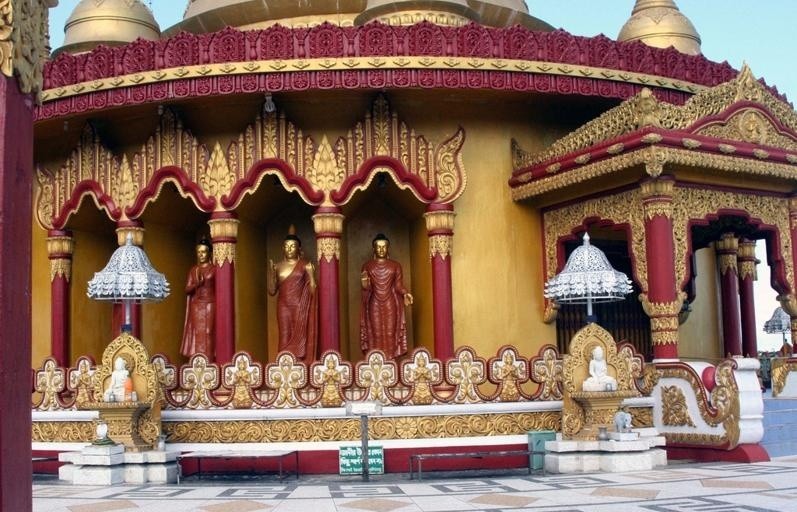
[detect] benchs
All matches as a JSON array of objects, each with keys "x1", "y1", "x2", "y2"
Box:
[
  {"x1": 176, "y1": 450, "x2": 300, "y2": 485},
  {"x1": 408, "y1": 449, "x2": 546, "y2": 483}
]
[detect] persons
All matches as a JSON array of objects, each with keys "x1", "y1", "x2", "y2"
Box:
[
  {"x1": 178, "y1": 234, "x2": 216, "y2": 361},
  {"x1": 501, "y1": 351, "x2": 519, "y2": 396},
  {"x1": 74, "y1": 360, "x2": 91, "y2": 405},
  {"x1": 266, "y1": 224, "x2": 317, "y2": 361},
  {"x1": 359, "y1": 232, "x2": 414, "y2": 361},
  {"x1": 586, "y1": 345, "x2": 614, "y2": 384},
  {"x1": 781, "y1": 338, "x2": 792, "y2": 353},
  {"x1": 414, "y1": 352, "x2": 431, "y2": 400},
  {"x1": 232, "y1": 356, "x2": 251, "y2": 403},
  {"x1": 103, "y1": 357, "x2": 132, "y2": 402},
  {"x1": 323, "y1": 356, "x2": 341, "y2": 401},
  {"x1": 153, "y1": 358, "x2": 169, "y2": 402}
]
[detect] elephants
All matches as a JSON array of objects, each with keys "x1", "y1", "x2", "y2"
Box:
[{"x1": 613, "y1": 410, "x2": 635, "y2": 434}]
